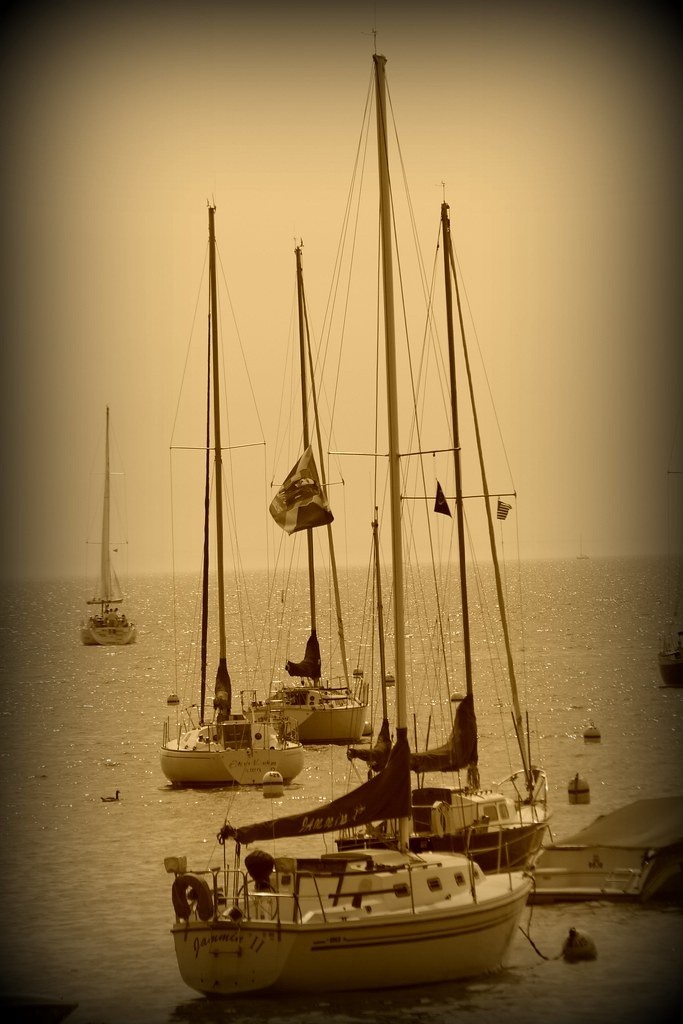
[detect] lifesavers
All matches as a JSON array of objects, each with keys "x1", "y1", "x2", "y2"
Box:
[
  {"x1": 431, "y1": 800, "x2": 457, "y2": 837},
  {"x1": 169, "y1": 873, "x2": 215, "y2": 922}
]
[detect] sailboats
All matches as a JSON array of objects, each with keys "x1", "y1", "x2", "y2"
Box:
[
  {"x1": 655, "y1": 438, "x2": 683, "y2": 686},
  {"x1": 73, "y1": 402, "x2": 142, "y2": 648},
  {"x1": 142, "y1": 45, "x2": 557, "y2": 1003}
]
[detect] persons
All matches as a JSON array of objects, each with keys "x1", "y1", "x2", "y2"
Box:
[{"x1": 90, "y1": 604, "x2": 127, "y2": 627}]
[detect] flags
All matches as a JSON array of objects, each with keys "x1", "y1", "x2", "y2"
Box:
[
  {"x1": 434, "y1": 482, "x2": 451, "y2": 517},
  {"x1": 497, "y1": 499, "x2": 512, "y2": 520},
  {"x1": 268, "y1": 446, "x2": 334, "y2": 536}
]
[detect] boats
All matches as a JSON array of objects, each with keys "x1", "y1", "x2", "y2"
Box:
[{"x1": 526, "y1": 793, "x2": 683, "y2": 904}]
